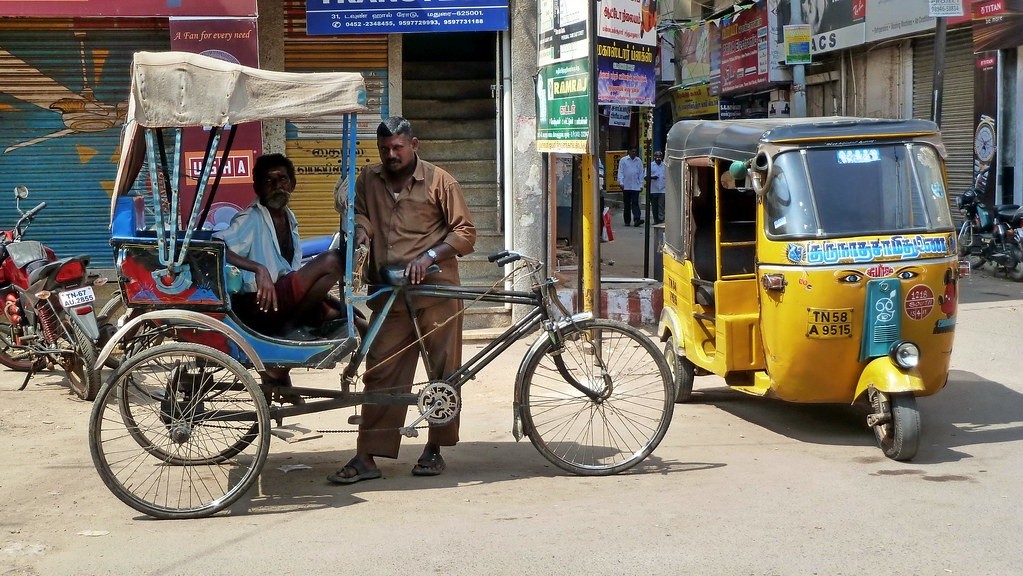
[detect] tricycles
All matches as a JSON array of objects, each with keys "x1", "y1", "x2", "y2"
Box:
[{"x1": 87, "y1": 50, "x2": 675, "y2": 519}]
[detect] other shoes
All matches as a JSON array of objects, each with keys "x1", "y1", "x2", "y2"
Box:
[
  {"x1": 625, "y1": 220, "x2": 630, "y2": 226},
  {"x1": 634, "y1": 219, "x2": 644, "y2": 227},
  {"x1": 652, "y1": 222, "x2": 658, "y2": 225}
]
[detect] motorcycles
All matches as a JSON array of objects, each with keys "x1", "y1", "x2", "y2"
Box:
[
  {"x1": 656, "y1": 117, "x2": 969, "y2": 461},
  {"x1": 0, "y1": 186, "x2": 164, "y2": 401}
]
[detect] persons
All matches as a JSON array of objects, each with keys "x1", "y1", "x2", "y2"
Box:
[
  {"x1": 644, "y1": 151, "x2": 666, "y2": 224},
  {"x1": 211, "y1": 153, "x2": 343, "y2": 337},
  {"x1": 617, "y1": 144, "x2": 644, "y2": 227},
  {"x1": 327, "y1": 116, "x2": 477, "y2": 483}
]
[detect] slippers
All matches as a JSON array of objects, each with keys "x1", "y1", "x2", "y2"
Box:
[
  {"x1": 330, "y1": 456, "x2": 382, "y2": 483},
  {"x1": 412, "y1": 448, "x2": 446, "y2": 474},
  {"x1": 258, "y1": 371, "x2": 305, "y2": 406},
  {"x1": 273, "y1": 328, "x2": 318, "y2": 340}
]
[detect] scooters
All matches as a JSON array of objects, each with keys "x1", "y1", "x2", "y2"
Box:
[{"x1": 957, "y1": 166, "x2": 1023, "y2": 281}]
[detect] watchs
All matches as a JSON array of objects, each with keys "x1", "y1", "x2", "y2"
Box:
[
  {"x1": 973, "y1": 114, "x2": 997, "y2": 193},
  {"x1": 427, "y1": 249, "x2": 438, "y2": 261}
]
